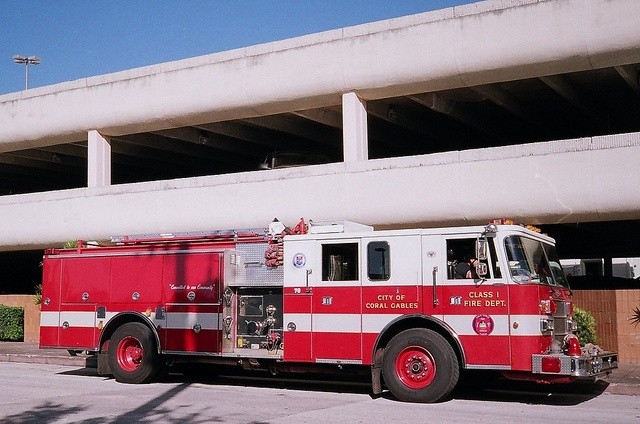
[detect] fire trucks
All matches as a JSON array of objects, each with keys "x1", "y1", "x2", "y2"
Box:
[{"x1": 39, "y1": 218, "x2": 619, "y2": 403}]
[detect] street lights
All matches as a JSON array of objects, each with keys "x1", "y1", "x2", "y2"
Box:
[{"x1": 14, "y1": 56, "x2": 40, "y2": 90}]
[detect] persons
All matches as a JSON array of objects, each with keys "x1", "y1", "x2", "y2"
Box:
[{"x1": 454, "y1": 247, "x2": 477, "y2": 278}]
